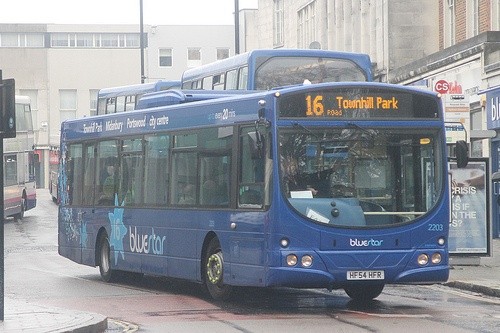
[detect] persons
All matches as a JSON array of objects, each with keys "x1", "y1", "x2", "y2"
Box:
[
  {"x1": 281, "y1": 158, "x2": 317, "y2": 197},
  {"x1": 176, "y1": 181, "x2": 218, "y2": 204}
]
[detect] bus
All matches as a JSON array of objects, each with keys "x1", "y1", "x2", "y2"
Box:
[
  {"x1": 179, "y1": 48, "x2": 376, "y2": 92},
  {"x1": 56, "y1": 78, "x2": 470, "y2": 303},
  {"x1": 95, "y1": 80, "x2": 182, "y2": 117},
  {"x1": 3, "y1": 96, "x2": 37, "y2": 222}
]
